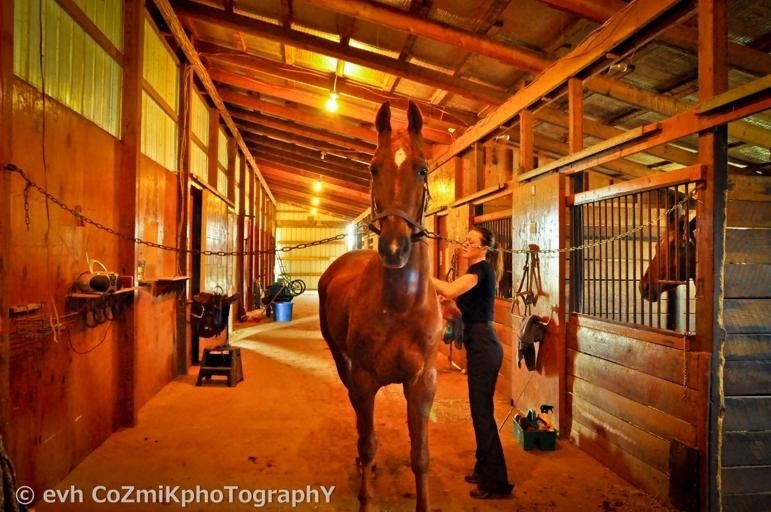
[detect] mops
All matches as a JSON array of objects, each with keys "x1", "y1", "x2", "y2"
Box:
[{"x1": 239, "y1": 280, "x2": 289, "y2": 323}]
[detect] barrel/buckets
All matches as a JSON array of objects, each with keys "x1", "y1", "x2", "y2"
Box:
[{"x1": 272, "y1": 301, "x2": 295, "y2": 323}]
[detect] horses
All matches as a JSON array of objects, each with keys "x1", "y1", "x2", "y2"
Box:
[
  {"x1": 637, "y1": 206, "x2": 697, "y2": 304},
  {"x1": 316, "y1": 99, "x2": 447, "y2": 512}
]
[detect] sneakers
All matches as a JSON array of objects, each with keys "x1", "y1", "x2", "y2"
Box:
[{"x1": 464, "y1": 472, "x2": 491, "y2": 498}]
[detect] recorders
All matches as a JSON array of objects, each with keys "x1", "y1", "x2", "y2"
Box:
[{"x1": 79, "y1": 260, "x2": 122, "y2": 294}]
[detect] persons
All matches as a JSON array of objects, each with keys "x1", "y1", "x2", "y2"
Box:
[{"x1": 432, "y1": 227, "x2": 509, "y2": 499}]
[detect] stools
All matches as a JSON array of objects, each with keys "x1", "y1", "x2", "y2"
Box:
[{"x1": 196, "y1": 345, "x2": 242, "y2": 387}]
